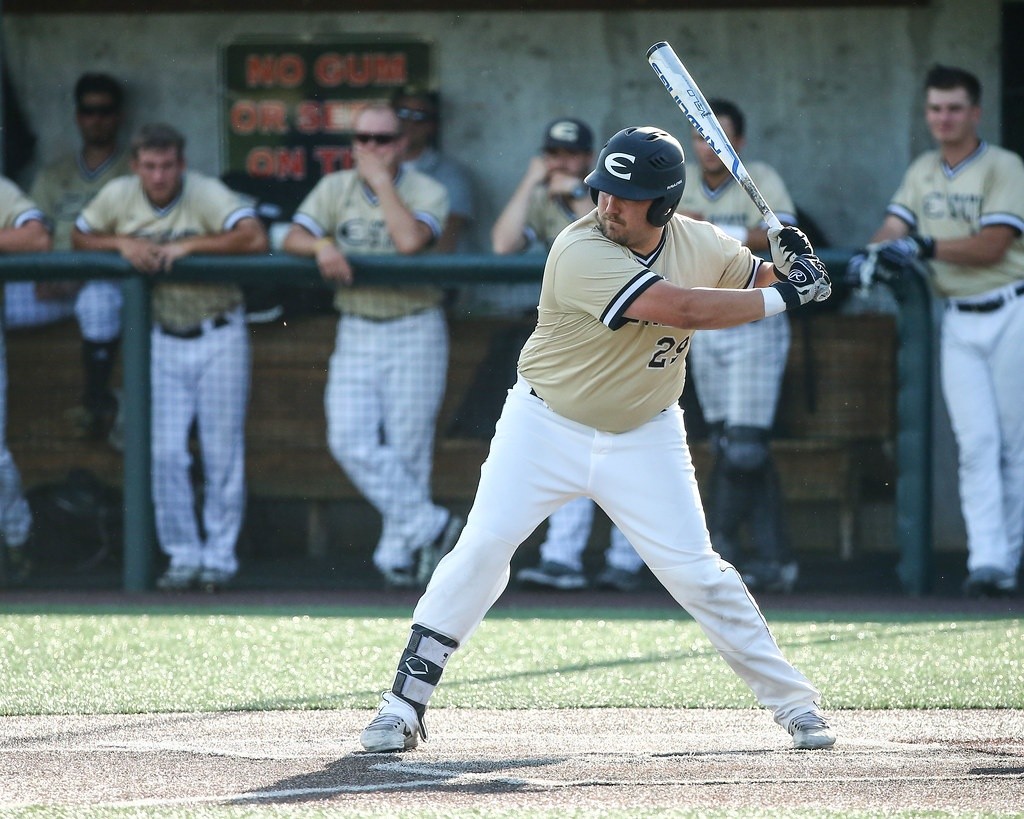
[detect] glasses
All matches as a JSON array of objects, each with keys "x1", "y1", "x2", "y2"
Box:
[
  {"x1": 543, "y1": 147, "x2": 583, "y2": 155},
  {"x1": 352, "y1": 131, "x2": 400, "y2": 145},
  {"x1": 395, "y1": 107, "x2": 434, "y2": 123},
  {"x1": 78, "y1": 105, "x2": 118, "y2": 116}
]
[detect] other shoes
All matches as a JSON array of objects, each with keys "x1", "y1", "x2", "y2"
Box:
[
  {"x1": 415, "y1": 513, "x2": 466, "y2": 590},
  {"x1": 384, "y1": 566, "x2": 417, "y2": 592},
  {"x1": 593, "y1": 565, "x2": 644, "y2": 593},
  {"x1": 759, "y1": 560, "x2": 800, "y2": 596},
  {"x1": 959, "y1": 566, "x2": 1020, "y2": 600},
  {"x1": 153, "y1": 566, "x2": 201, "y2": 592},
  {"x1": 516, "y1": 561, "x2": 590, "y2": 593},
  {"x1": 194, "y1": 567, "x2": 234, "y2": 593}
]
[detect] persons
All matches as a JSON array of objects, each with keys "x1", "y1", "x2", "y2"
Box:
[
  {"x1": 0, "y1": 74, "x2": 271, "y2": 590},
  {"x1": 284, "y1": 86, "x2": 464, "y2": 586},
  {"x1": 491, "y1": 101, "x2": 797, "y2": 593},
  {"x1": 361, "y1": 128, "x2": 837, "y2": 752},
  {"x1": 844, "y1": 63, "x2": 1024, "y2": 594}
]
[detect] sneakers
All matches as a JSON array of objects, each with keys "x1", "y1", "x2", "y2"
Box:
[
  {"x1": 359, "y1": 690, "x2": 430, "y2": 754},
  {"x1": 787, "y1": 709, "x2": 836, "y2": 751}
]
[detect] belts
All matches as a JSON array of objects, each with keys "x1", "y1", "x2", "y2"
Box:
[
  {"x1": 944, "y1": 284, "x2": 1024, "y2": 316},
  {"x1": 161, "y1": 314, "x2": 228, "y2": 339}
]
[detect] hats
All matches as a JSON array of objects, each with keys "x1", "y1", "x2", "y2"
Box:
[{"x1": 539, "y1": 117, "x2": 592, "y2": 152}]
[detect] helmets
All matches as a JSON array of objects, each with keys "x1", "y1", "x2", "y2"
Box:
[{"x1": 582, "y1": 126, "x2": 686, "y2": 227}]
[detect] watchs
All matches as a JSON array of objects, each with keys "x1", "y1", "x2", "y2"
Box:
[{"x1": 568, "y1": 182, "x2": 588, "y2": 199}]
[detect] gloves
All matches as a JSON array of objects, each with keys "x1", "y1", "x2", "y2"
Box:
[
  {"x1": 770, "y1": 253, "x2": 832, "y2": 311},
  {"x1": 766, "y1": 225, "x2": 814, "y2": 283},
  {"x1": 845, "y1": 236, "x2": 925, "y2": 291}
]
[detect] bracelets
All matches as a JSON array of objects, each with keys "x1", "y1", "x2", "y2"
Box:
[{"x1": 314, "y1": 237, "x2": 332, "y2": 254}]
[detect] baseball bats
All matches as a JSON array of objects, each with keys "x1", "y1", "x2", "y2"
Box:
[{"x1": 645, "y1": 40, "x2": 831, "y2": 302}]
